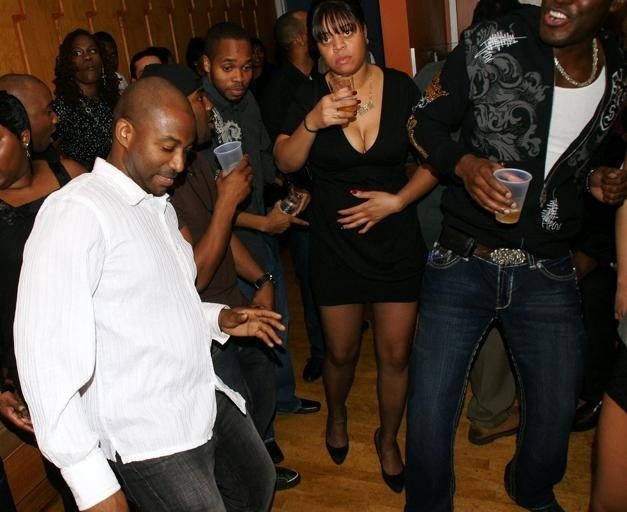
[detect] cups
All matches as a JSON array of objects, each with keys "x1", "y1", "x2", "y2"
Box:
[
  {"x1": 328, "y1": 75, "x2": 357, "y2": 117},
  {"x1": 213, "y1": 142, "x2": 244, "y2": 175},
  {"x1": 491, "y1": 168, "x2": 532, "y2": 223}
]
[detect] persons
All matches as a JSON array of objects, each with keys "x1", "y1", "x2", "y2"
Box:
[{"x1": 0, "y1": 0, "x2": 627, "y2": 510}]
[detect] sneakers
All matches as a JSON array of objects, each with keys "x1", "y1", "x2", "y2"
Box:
[{"x1": 468, "y1": 405, "x2": 520, "y2": 445}]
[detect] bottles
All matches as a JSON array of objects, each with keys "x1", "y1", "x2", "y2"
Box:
[{"x1": 280, "y1": 188, "x2": 304, "y2": 216}]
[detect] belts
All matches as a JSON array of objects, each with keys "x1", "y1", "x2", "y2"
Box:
[{"x1": 438, "y1": 222, "x2": 573, "y2": 268}]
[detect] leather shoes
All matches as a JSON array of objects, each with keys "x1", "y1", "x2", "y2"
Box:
[
  {"x1": 374, "y1": 428, "x2": 405, "y2": 493},
  {"x1": 264, "y1": 436, "x2": 300, "y2": 491},
  {"x1": 276, "y1": 398, "x2": 321, "y2": 414},
  {"x1": 326, "y1": 436, "x2": 349, "y2": 465},
  {"x1": 303, "y1": 356, "x2": 325, "y2": 382},
  {"x1": 572, "y1": 393, "x2": 601, "y2": 431}
]
